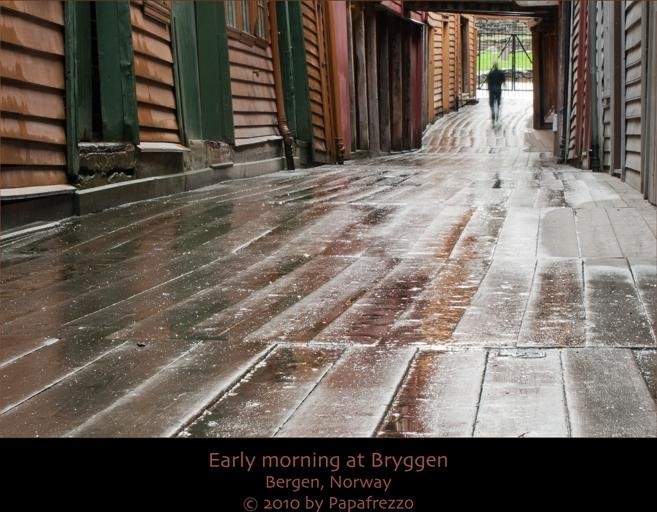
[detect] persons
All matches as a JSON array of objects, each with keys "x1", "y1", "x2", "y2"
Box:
[{"x1": 486, "y1": 61, "x2": 506, "y2": 129}]
